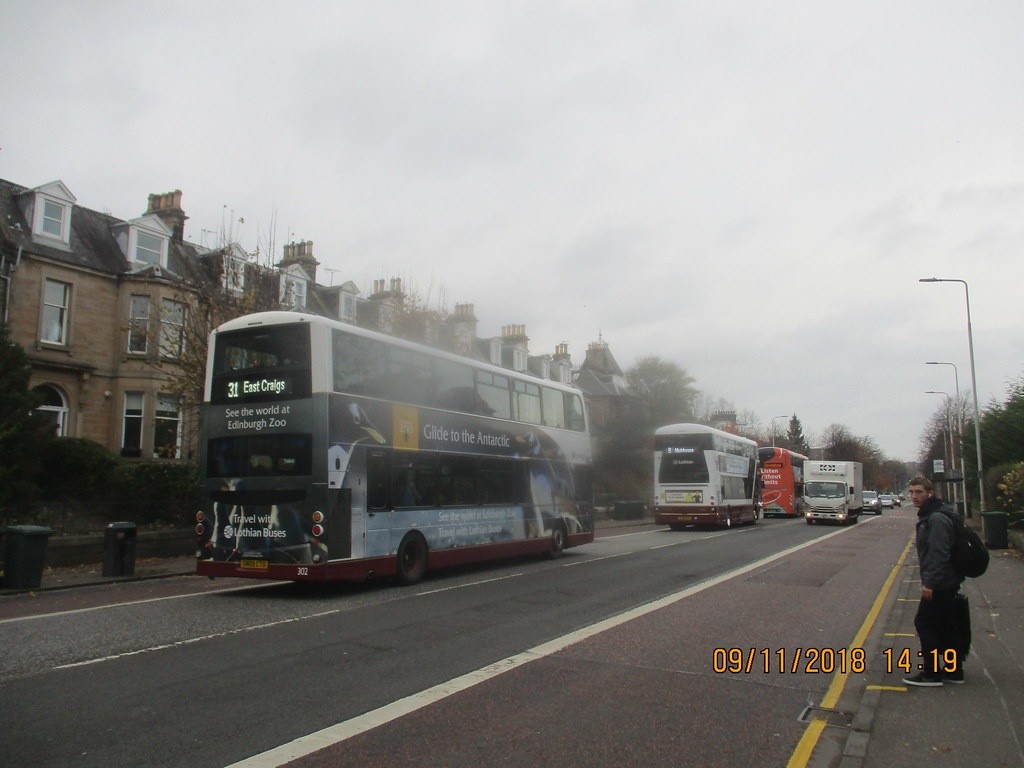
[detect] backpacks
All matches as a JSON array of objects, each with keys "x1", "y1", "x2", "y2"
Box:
[{"x1": 956, "y1": 520, "x2": 990, "y2": 578}]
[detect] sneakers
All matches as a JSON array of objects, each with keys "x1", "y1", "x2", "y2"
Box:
[
  {"x1": 902, "y1": 670, "x2": 944, "y2": 688},
  {"x1": 942, "y1": 669, "x2": 966, "y2": 685}
]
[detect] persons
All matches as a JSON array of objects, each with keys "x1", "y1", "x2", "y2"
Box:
[
  {"x1": 394, "y1": 468, "x2": 422, "y2": 507},
  {"x1": 901, "y1": 474, "x2": 972, "y2": 687}
]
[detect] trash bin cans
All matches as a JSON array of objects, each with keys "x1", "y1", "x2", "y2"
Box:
[
  {"x1": 104, "y1": 520, "x2": 137, "y2": 577},
  {"x1": 4, "y1": 526, "x2": 56, "y2": 588},
  {"x1": 981, "y1": 510, "x2": 1008, "y2": 549},
  {"x1": 956, "y1": 499, "x2": 972, "y2": 518},
  {"x1": 615, "y1": 500, "x2": 644, "y2": 520}
]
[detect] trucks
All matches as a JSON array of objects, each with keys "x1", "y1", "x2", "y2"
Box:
[{"x1": 803, "y1": 460, "x2": 864, "y2": 525}]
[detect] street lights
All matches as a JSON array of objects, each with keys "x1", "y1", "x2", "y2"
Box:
[
  {"x1": 925, "y1": 361, "x2": 968, "y2": 518},
  {"x1": 924, "y1": 391, "x2": 959, "y2": 512},
  {"x1": 929, "y1": 427, "x2": 950, "y2": 502},
  {"x1": 919, "y1": 277, "x2": 989, "y2": 546}
]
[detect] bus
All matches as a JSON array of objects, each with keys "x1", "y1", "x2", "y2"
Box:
[
  {"x1": 655, "y1": 420, "x2": 761, "y2": 529},
  {"x1": 195, "y1": 308, "x2": 596, "y2": 585},
  {"x1": 756, "y1": 445, "x2": 810, "y2": 518}
]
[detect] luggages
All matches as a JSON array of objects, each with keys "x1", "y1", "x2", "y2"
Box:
[{"x1": 914, "y1": 591, "x2": 972, "y2": 663}]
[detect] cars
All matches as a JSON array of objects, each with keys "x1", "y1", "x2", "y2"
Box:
[
  {"x1": 881, "y1": 496, "x2": 894, "y2": 511},
  {"x1": 894, "y1": 474, "x2": 915, "y2": 502},
  {"x1": 893, "y1": 495, "x2": 901, "y2": 506},
  {"x1": 862, "y1": 490, "x2": 882, "y2": 517}
]
